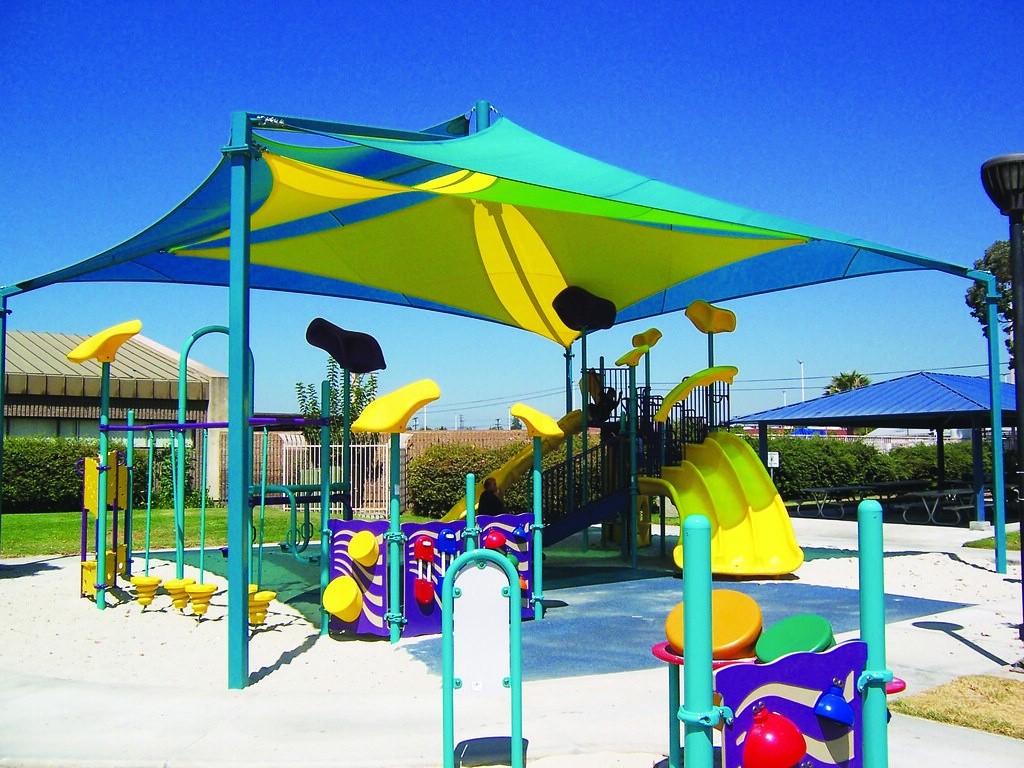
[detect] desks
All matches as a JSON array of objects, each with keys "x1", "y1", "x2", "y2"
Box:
[{"x1": 800, "y1": 479, "x2": 1020, "y2": 525}]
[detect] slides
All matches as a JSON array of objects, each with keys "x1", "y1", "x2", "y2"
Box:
[{"x1": 637, "y1": 430, "x2": 804, "y2": 576}]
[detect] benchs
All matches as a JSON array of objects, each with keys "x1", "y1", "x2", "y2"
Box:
[{"x1": 797, "y1": 489, "x2": 1009, "y2": 525}]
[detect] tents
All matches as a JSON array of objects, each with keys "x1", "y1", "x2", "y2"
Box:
[
  {"x1": 727, "y1": 370, "x2": 1024, "y2": 520},
  {"x1": 0, "y1": 101, "x2": 1008, "y2": 687}
]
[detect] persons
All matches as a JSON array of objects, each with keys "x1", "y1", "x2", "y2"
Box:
[
  {"x1": 479, "y1": 478, "x2": 506, "y2": 516},
  {"x1": 587, "y1": 369, "x2": 623, "y2": 422}
]
